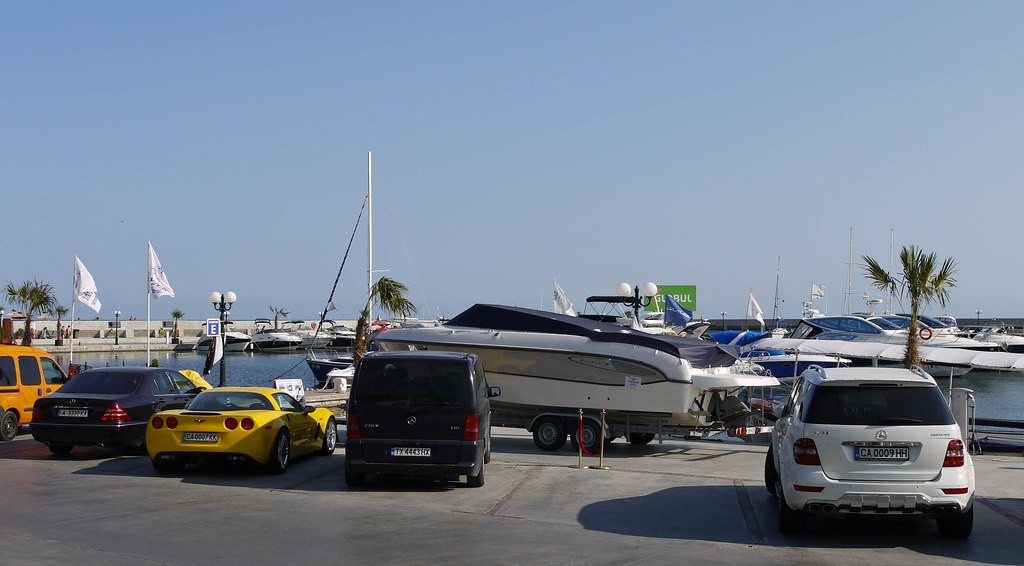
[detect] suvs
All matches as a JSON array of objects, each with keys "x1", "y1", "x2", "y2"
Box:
[{"x1": 764, "y1": 363, "x2": 976, "y2": 542}]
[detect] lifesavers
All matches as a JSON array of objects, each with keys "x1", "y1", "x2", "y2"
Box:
[{"x1": 919, "y1": 327, "x2": 932, "y2": 341}]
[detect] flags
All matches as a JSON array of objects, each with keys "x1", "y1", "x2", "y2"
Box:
[
  {"x1": 747, "y1": 293, "x2": 765, "y2": 326},
  {"x1": 74, "y1": 255, "x2": 102, "y2": 313},
  {"x1": 664, "y1": 294, "x2": 693, "y2": 328},
  {"x1": 147, "y1": 241, "x2": 175, "y2": 298},
  {"x1": 553, "y1": 279, "x2": 578, "y2": 317},
  {"x1": 812, "y1": 283, "x2": 826, "y2": 299}
]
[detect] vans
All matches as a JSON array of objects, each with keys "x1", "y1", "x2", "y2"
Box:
[{"x1": 342, "y1": 351, "x2": 501, "y2": 488}]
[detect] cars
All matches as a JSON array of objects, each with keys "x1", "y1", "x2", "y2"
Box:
[
  {"x1": 0, "y1": 343, "x2": 71, "y2": 445},
  {"x1": 29, "y1": 366, "x2": 208, "y2": 454},
  {"x1": 145, "y1": 382, "x2": 337, "y2": 481}
]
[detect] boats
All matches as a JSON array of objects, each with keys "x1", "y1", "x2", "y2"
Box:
[
  {"x1": 296, "y1": 329, "x2": 336, "y2": 351},
  {"x1": 305, "y1": 353, "x2": 354, "y2": 385},
  {"x1": 372, "y1": 303, "x2": 697, "y2": 451},
  {"x1": 326, "y1": 326, "x2": 366, "y2": 345},
  {"x1": 252, "y1": 329, "x2": 303, "y2": 351},
  {"x1": 192, "y1": 331, "x2": 252, "y2": 352}
]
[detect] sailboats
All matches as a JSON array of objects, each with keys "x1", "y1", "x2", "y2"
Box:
[{"x1": 686, "y1": 224, "x2": 1023, "y2": 384}]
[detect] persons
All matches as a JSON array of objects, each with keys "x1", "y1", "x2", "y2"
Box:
[{"x1": 61, "y1": 325, "x2": 71, "y2": 339}]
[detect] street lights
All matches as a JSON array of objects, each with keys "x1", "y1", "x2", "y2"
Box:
[
  {"x1": 0, "y1": 309, "x2": 5, "y2": 337},
  {"x1": 211, "y1": 290, "x2": 236, "y2": 387},
  {"x1": 114, "y1": 310, "x2": 122, "y2": 344},
  {"x1": 975, "y1": 308, "x2": 983, "y2": 326},
  {"x1": 617, "y1": 282, "x2": 658, "y2": 328}
]
